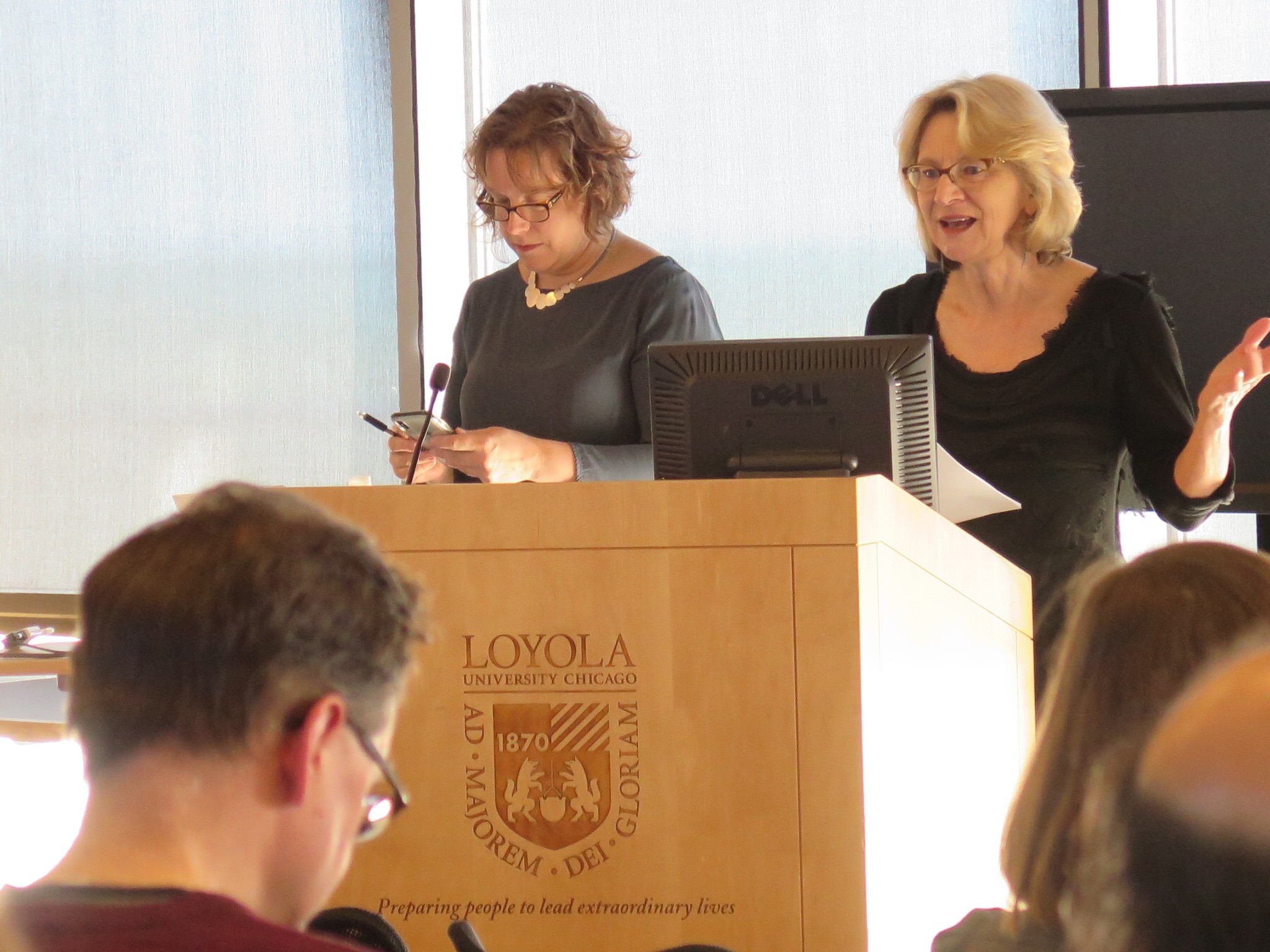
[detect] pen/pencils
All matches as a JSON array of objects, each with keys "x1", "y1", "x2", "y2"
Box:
[{"x1": 356, "y1": 411, "x2": 402, "y2": 438}]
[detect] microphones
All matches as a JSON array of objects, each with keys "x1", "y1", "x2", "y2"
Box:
[{"x1": 406, "y1": 362, "x2": 451, "y2": 485}]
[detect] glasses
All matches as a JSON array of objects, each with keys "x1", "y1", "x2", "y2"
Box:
[
  {"x1": 293, "y1": 696, "x2": 411, "y2": 841},
  {"x1": 475, "y1": 176, "x2": 572, "y2": 222},
  {"x1": 900, "y1": 154, "x2": 998, "y2": 191}
]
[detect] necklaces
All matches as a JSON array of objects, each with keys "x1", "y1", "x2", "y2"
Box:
[{"x1": 524, "y1": 220, "x2": 615, "y2": 308}]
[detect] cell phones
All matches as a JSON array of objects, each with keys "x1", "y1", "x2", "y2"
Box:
[{"x1": 391, "y1": 411, "x2": 457, "y2": 437}]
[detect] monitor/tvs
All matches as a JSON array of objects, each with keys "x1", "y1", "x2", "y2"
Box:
[
  {"x1": 1033, "y1": 84, "x2": 1270, "y2": 515},
  {"x1": 646, "y1": 334, "x2": 938, "y2": 514}
]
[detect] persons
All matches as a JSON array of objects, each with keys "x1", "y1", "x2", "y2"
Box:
[
  {"x1": 867, "y1": 72, "x2": 1270, "y2": 707},
  {"x1": 0, "y1": 482, "x2": 431, "y2": 952},
  {"x1": 931, "y1": 540, "x2": 1270, "y2": 952},
  {"x1": 387, "y1": 80, "x2": 728, "y2": 488}
]
